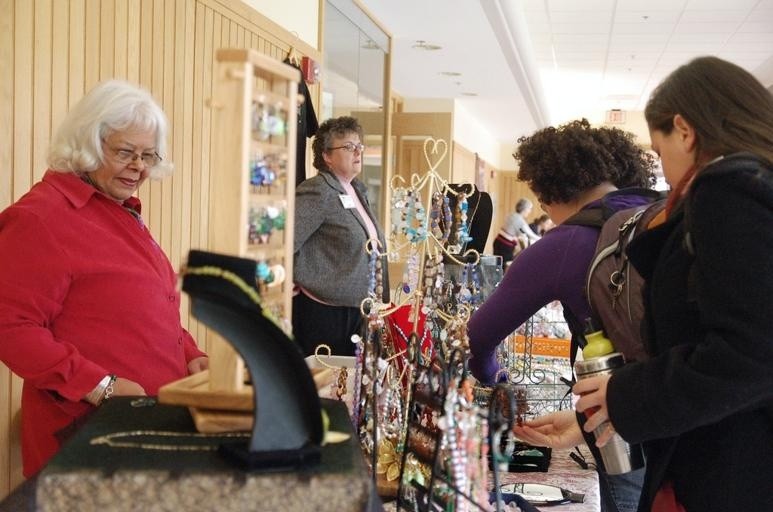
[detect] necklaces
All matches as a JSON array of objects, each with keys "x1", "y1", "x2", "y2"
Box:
[
  {"x1": 336, "y1": 311, "x2": 492, "y2": 511},
  {"x1": 179, "y1": 262, "x2": 276, "y2": 324},
  {"x1": 90, "y1": 428, "x2": 352, "y2": 452}
]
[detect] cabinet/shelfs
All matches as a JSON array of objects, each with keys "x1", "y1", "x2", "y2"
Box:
[{"x1": 208, "y1": 45, "x2": 309, "y2": 393}]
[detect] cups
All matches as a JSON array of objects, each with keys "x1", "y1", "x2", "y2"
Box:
[{"x1": 573, "y1": 354, "x2": 645, "y2": 477}]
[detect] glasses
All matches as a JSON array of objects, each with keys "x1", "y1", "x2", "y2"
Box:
[
  {"x1": 100, "y1": 138, "x2": 162, "y2": 170},
  {"x1": 329, "y1": 144, "x2": 365, "y2": 152}
]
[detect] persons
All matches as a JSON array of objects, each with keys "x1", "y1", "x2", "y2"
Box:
[
  {"x1": 1, "y1": 78, "x2": 209, "y2": 481},
  {"x1": 466, "y1": 118, "x2": 672, "y2": 512},
  {"x1": 293, "y1": 116, "x2": 386, "y2": 357},
  {"x1": 493, "y1": 199, "x2": 542, "y2": 276},
  {"x1": 519, "y1": 214, "x2": 550, "y2": 249},
  {"x1": 511, "y1": 56, "x2": 773, "y2": 511}
]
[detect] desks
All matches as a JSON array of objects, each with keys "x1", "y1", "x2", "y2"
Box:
[
  {"x1": 156, "y1": 371, "x2": 254, "y2": 415},
  {"x1": 477, "y1": 436, "x2": 604, "y2": 512}
]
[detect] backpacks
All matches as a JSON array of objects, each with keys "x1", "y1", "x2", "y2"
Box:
[{"x1": 561, "y1": 190, "x2": 668, "y2": 364}]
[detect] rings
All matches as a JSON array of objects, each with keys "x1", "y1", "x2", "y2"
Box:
[{"x1": 607, "y1": 425, "x2": 616, "y2": 435}]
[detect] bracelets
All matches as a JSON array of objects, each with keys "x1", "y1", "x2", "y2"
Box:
[
  {"x1": 367, "y1": 186, "x2": 481, "y2": 314},
  {"x1": 130, "y1": 397, "x2": 156, "y2": 409},
  {"x1": 101, "y1": 374, "x2": 115, "y2": 404}
]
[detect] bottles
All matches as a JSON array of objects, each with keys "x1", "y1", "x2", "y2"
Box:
[
  {"x1": 476, "y1": 257, "x2": 503, "y2": 305},
  {"x1": 247, "y1": 97, "x2": 286, "y2": 246},
  {"x1": 582, "y1": 317, "x2": 614, "y2": 359}
]
